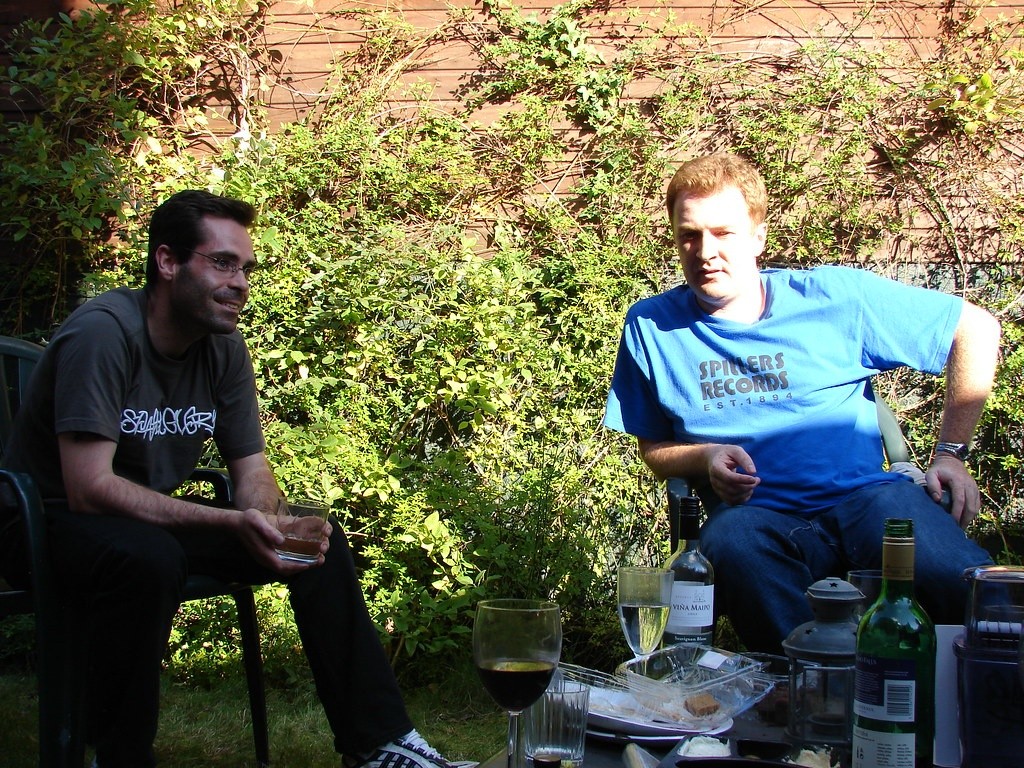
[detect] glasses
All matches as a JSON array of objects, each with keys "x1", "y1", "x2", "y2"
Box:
[{"x1": 172, "y1": 245, "x2": 260, "y2": 285}]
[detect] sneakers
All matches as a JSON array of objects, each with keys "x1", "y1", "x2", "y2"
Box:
[{"x1": 343, "y1": 728, "x2": 479, "y2": 768}]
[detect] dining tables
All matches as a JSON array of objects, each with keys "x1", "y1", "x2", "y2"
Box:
[{"x1": 476, "y1": 720, "x2": 1023, "y2": 768}]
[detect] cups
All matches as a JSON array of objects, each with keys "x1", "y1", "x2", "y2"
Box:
[
  {"x1": 523, "y1": 681, "x2": 590, "y2": 768},
  {"x1": 273, "y1": 498, "x2": 330, "y2": 565},
  {"x1": 847, "y1": 570, "x2": 882, "y2": 625}
]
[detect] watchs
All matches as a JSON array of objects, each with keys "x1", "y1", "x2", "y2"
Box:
[{"x1": 936, "y1": 443, "x2": 969, "y2": 461}]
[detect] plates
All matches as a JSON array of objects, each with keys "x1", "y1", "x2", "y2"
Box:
[{"x1": 544, "y1": 678, "x2": 733, "y2": 743}]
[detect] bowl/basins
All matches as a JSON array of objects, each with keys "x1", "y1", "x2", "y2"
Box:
[
  {"x1": 618, "y1": 642, "x2": 760, "y2": 726},
  {"x1": 738, "y1": 651, "x2": 823, "y2": 715}
]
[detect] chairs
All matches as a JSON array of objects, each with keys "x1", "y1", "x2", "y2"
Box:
[
  {"x1": 0, "y1": 336, "x2": 268, "y2": 768},
  {"x1": 665, "y1": 393, "x2": 953, "y2": 652}
]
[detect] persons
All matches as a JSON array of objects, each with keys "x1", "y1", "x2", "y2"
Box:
[
  {"x1": 2, "y1": 191, "x2": 480, "y2": 767},
  {"x1": 603, "y1": 153, "x2": 1000, "y2": 674}
]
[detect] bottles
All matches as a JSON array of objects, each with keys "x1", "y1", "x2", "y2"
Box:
[
  {"x1": 850, "y1": 517, "x2": 938, "y2": 768},
  {"x1": 659, "y1": 497, "x2": 715, "y2": 676}
]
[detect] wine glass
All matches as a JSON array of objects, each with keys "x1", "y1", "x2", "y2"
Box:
[
  {"x1": 471, "y1": 598, "x2": 563, "y2": 768},
  {"x1": 617, "y1": 567, "x2": 674, "y2": 676}
]
